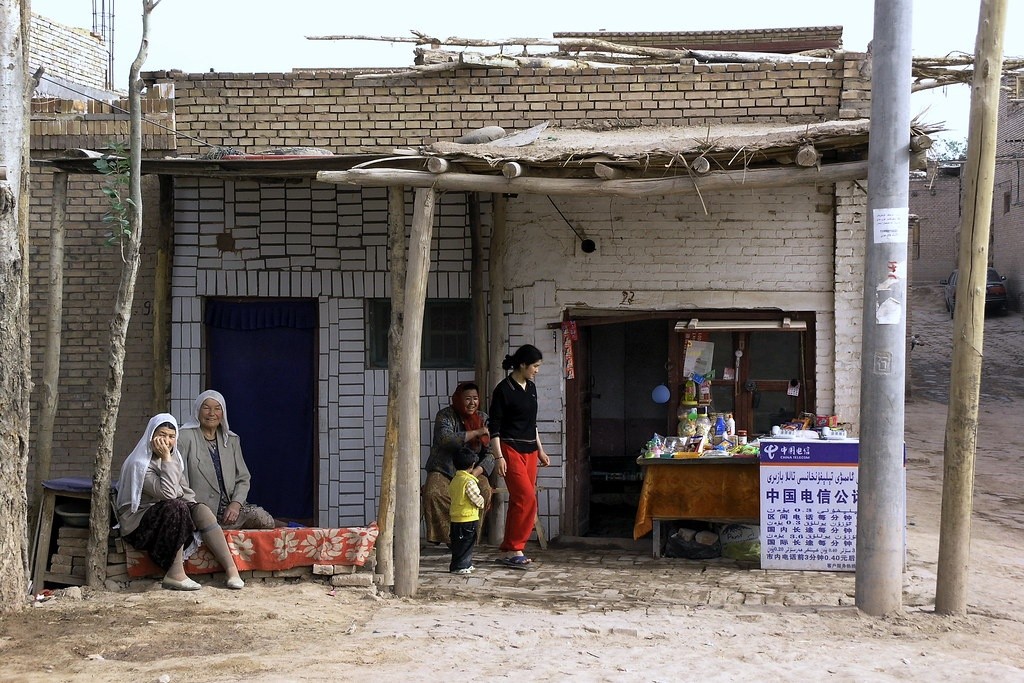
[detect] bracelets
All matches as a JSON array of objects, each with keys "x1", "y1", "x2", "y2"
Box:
[{"x1": 494, "y1": 455, "x2": 503, "y2": 460}]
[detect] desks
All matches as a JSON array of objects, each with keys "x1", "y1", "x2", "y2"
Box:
[{"x1": 637, "y1": 431, "x2": 759, "y2": 561}]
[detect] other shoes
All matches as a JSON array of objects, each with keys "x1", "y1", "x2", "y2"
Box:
[
  {"x1": 225, "y1": 575, "x2": 244, "y2": 587},
  {"x1": 162, "y1": 575, "x2": 202, "y2": 591}
]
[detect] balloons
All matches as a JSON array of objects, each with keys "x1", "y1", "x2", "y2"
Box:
[{"x1": 652, "y1": 383, "x2": 670, "y2": 403}]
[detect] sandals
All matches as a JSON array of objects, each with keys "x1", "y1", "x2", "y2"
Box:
[
  {"x1": 452, "y1": 569, "x2": 471, "y2": 575},
  {"x1": 468, "y1": 566, "x2": 476, "y2": 570}
]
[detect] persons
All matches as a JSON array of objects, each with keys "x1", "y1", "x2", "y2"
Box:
[
  {"x1": 423, "y1": 381, "x2": 496, "y2": 546},
  {"x1": 117, "y1": 413, "x2": 245, "y2": 591},
  {"x1": 177, "y1": 390, "x2": 311, "y2": 529},
  {"x1": 448, "y1": 447, "x2": 486, "y2": 574},
  {"x1": 490, "y1": 343, "x2": 551, "y2": 567}
]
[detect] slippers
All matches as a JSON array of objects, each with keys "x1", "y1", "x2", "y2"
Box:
[
  {"x1": 494, "y1": 559, "x2": 506, "y2": 565},
  {"x1": 502, "y1": 556, "x2": 534, "y2": 568}
]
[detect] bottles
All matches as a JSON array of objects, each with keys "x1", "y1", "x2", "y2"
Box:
[
  {"x1": 695, "y1": 412, "x2": 735, "y2": 448},
  {"x1": 481, "y1": 435, "x2": 489, "y2": 444},
  {"x1": 686, "y1": 377, "x2": 695, "y2": 401}
]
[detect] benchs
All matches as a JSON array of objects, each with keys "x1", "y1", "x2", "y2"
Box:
[
  {"x1": 493, "y1": 485, "x2": 548, "y2": 549},
  {"x1": 121, "y1": 526, "x2": 378, "y2": 571}
]
